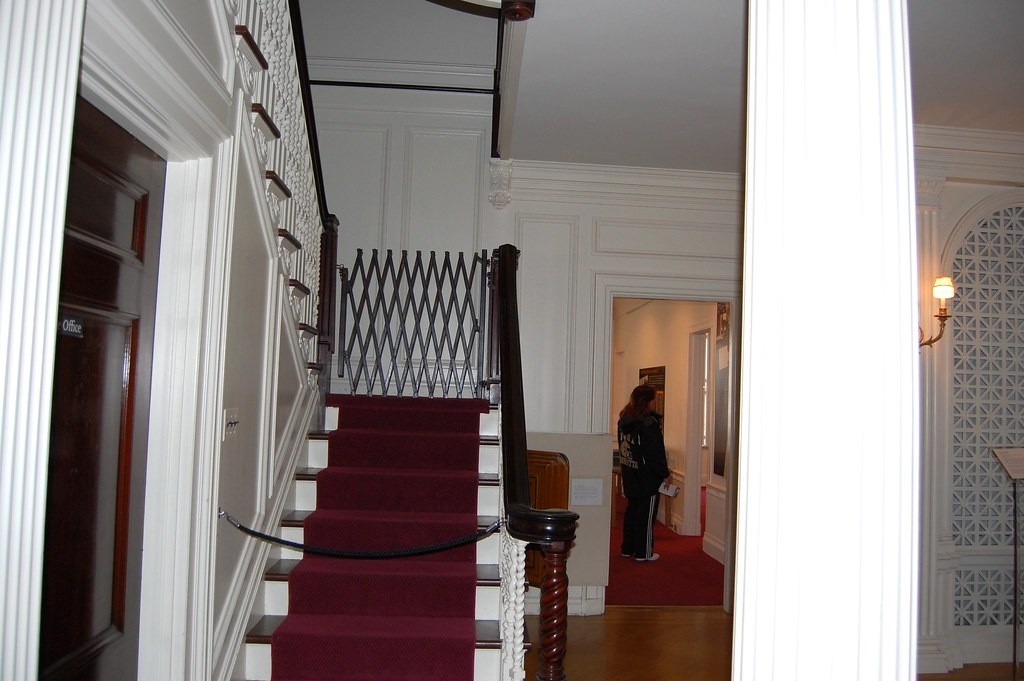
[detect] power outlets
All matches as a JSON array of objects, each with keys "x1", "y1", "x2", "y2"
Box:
[{"x1": 222, "y1": 407, "x2": 241, "y2": 442}]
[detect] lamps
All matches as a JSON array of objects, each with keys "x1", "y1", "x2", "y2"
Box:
[{"x1": 917, "y1": 276, "x2": 956, "y2": 347}]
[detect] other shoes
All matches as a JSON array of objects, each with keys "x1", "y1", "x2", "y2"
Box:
[
  {"x1": 622, "y1": 553, "x2": 635, "y2": 557},
  {"x1": 634, "y1": 553, "x2": 659, "y2": 561}
]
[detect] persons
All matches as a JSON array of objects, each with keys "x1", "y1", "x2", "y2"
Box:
[{"x1": 618, "y1": 384, "x2": 674, "y2": 562}]
